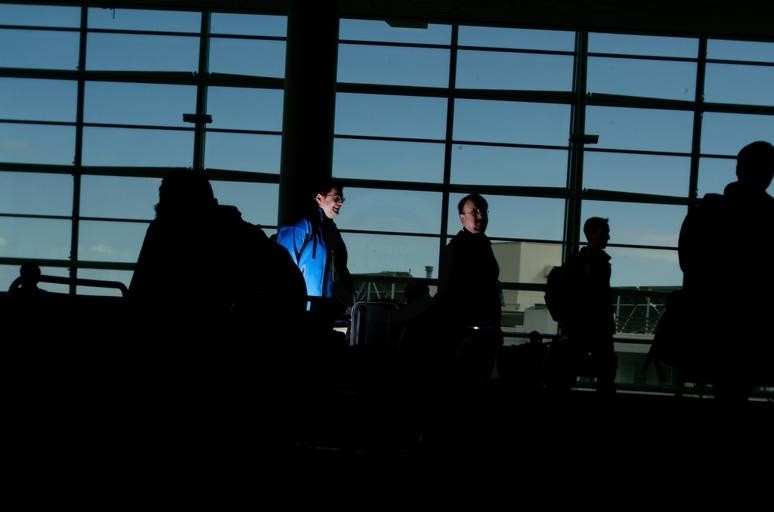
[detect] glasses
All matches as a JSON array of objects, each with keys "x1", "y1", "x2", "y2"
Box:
[
  {"x1": 462, "y1": 209, "x2": 489, "y2": 215},
  {"x1": 324, "y1": 195, "x2": 345, "y2": 201}
]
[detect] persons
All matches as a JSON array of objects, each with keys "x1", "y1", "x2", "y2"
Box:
[
  {"x1": 681, "y1": 140, "x2": 773, "y2": 403},
  {"x1": 555, "y1": 217, "x2": 619, "y2": 397},
  {"x1": 437, "y1": 190, "x2": 505, "y2": 345},
  {"x1": 116, "y1": 167, "x2": 247, "y2": 511},
  {"x1": 277, "y1": 176, "x2": 361, "y2": 336}
]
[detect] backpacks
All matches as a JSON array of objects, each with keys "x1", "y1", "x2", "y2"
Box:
[{"x1": 544, "y1": 266, "x2": 585, "y2": 324}]
